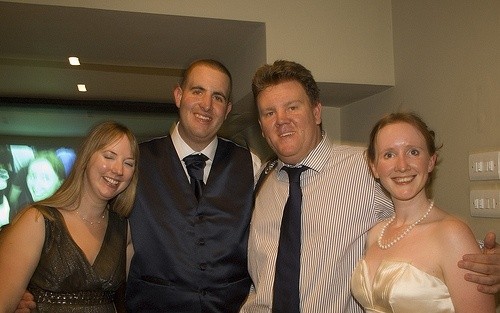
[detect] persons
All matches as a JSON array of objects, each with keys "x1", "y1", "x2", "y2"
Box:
[
  {"x1": 26, "y1": 150, "x2": 66, "y2": 203},
  {"x1": 350, "y1": 112, "x2": 496, "y2": 313},
  {"x1": 14, "y1": 59, "x2": 261, "y2": 313},
  {"x1": 0, "y1": 120, "x2": 141, "y2": 313},
  {"x1": 237, "y1": 60, "x2": 500, "y2": 313}
]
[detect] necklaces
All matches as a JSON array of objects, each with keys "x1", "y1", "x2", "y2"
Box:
[
  {"x1": 74, "y1": 210, "x2": 106, "y2": 225},
  {"x1": 377, "y1": 198, "x2": 435, "y2": 249}
]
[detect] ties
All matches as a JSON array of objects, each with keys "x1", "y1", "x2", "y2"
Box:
[
  {"x1": 182, "y1": 152, "x2": 210, "y2": 202},
  {"x1": 271, "y1": 166, "x2": 309, "y2": 313}
]
